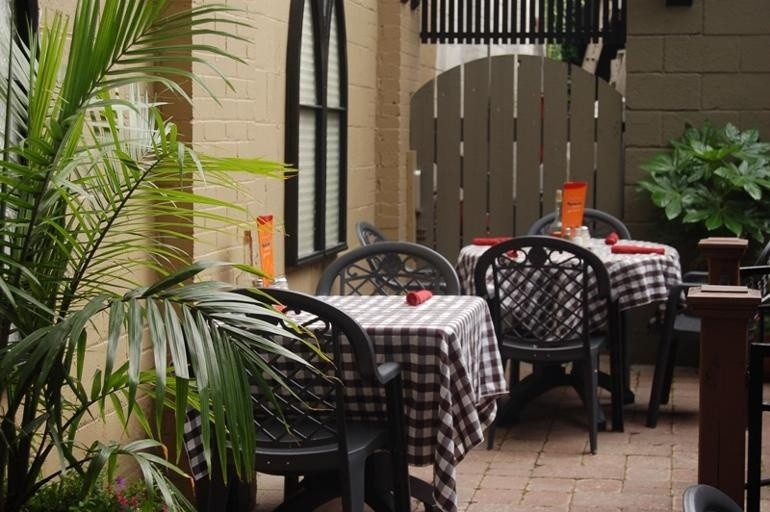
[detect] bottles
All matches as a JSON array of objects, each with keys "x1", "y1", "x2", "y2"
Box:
[
  {"x1": 549, "y1": 189, "x2": 562, "y2": 236},
  {"x1": 563, "y1": 226, "x2": 573, "y2": 240},
  {"x1": 575, "y1": 225, "x2": 592, "y2": 248},
  {"x1": 255, "y1": 277, "x2": 289, "y2": 292}
]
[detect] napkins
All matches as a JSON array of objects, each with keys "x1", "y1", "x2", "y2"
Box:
[
  {"x1": 610, "y1": 244, "x2": 665, "y2": 255},
  {"x1": 404, "y1": 290, "x2": 431, "y2": 307},
  {"x1": 472, "y1": 236, "x2": 516, "y2": 245},
  {"x1": 605, "y1": 231, "x2": 619, "y2": 245}
]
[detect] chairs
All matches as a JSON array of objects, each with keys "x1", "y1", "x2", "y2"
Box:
[
  {"x1": 177, "y1": 288, "x2": 412, "y2": 511},
  {"x1": 683, "y1": 485, "x2": 743, "y2": 511},
  {"x1": 314, "y1": 240, "x2": 463, "y2": 297},
  {"x1": 525, "y1": 207, "x2": 630, "y2": 240},
  {"x1": 473, "y1": 234, "x2": 624, "y2": 456},
  {"x1": 355, "y1": 220, "x2": 447, "y2": 294},
  {"x1": 647, "y1": 239, "x2": 769, "y2": 430}
]
